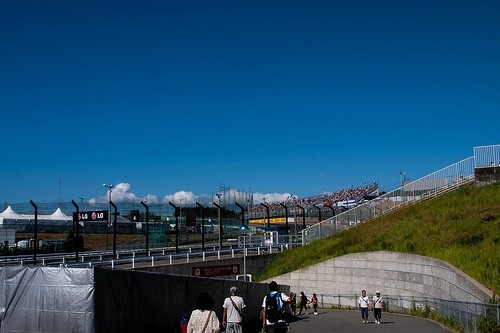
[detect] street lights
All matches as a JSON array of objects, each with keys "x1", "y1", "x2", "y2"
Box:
[
  {"x1": 29, "y1": 200, "x2": 38, "y2": 267},
  {"x1": 141, "y1": 200, "x2": 149, "y2": 256},
  {"x1": 260, "y1": 203, "x2": 270, "y2": 228},
  {"x1": 297, "y1": 204, "x2": 305, "y2": 230},
  {"x1": 328, "y1": 205, "x2": 335, "y2": 216},
  {"x1": 212, "y1": 202, "x2": 222, "y2": 249},
  {"x1": 71, "y1": 199, "x2": 80, "y2": 263},
  {"x1": 196, "y1": 201, "x2": 204, "y2": 257},
  {"x1": 234, "y1": 201, "x2": 245, "y2": 226},
  {"x1": 102, "y1": 183, "x2": 114, "y2": 228},
  {"x1": 109, "y1": 200, "x2": 117, "y2": 259},
  {"x1": 313, "y1": 204, "x2": 321, "y2": 223},
  {"x1": 280, "y1": 202, "x2": 288, "y2": 228},
  {"x1": 168, "y1": 201, "x2": 179, "y2": 254}
]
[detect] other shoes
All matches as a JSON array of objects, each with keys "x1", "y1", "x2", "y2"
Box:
[
  {"x1": 366, "y1": 320, "x2": 368, "y2": 324},
  {"x1": 378, "y1": 321, "x2": 381, "y2": 325},
  {"x1": 363, "y1": 320, "x2": 365, "y2": 324},
  {"x1": 374, "y1": 319, "x2": 377, "y2": 323}
]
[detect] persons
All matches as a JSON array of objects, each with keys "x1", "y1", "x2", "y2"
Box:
[
  {"x1": 298, "y1": 292, "x2": 308, "y2": 316},
  {"x1": 262, "y1": 281, "x2": 296, "y2": 333},
  {"x1": 47, "y1": 242, "x2": 51, "y2": 250},
  {"x1": 222, "y1": 287, "x2": 246, "y2": 333},
  {"x1": 186, "y1": 291, "x2": 220, "y2": 333},
  {"x1": 359, "y1": 290, "x2": 370, "y2": 324},
  {"x1": 41, "y1": 243, "x2": 47, "y2": 250},
  {"x1": 244, "y1": 180, "x2": 376, "y2": 213},
  {"x1": 373, "y1": 291, "x2": 383, "y2": 325},
  {"x1": 310, "y1": 293, "x2": 318, "y2": 315}
]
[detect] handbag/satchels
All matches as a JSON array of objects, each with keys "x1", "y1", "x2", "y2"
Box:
[{"x1": 241, "y1": 311, "x2": 248, "y2": 323}]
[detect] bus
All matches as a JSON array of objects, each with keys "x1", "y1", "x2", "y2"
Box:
[{"x1": 8, "y1": 238, "x2": 43, "y2": 251}]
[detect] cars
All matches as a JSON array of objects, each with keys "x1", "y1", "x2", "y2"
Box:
[{"x1": 323, "y1": 199, "x2": 369, "y2": 209}]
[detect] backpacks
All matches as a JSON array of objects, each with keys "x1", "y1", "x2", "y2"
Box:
[{"x1": 266, "y1": 292, "x2": 284, "y2": 323}]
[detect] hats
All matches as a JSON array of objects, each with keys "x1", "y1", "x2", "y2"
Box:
[
  {"x1": 376, "y1": 291, "x2": 380, "y2": 294},
  {"x1": 230, "y1": 286, "x2": 237, "y2": 293}
]
